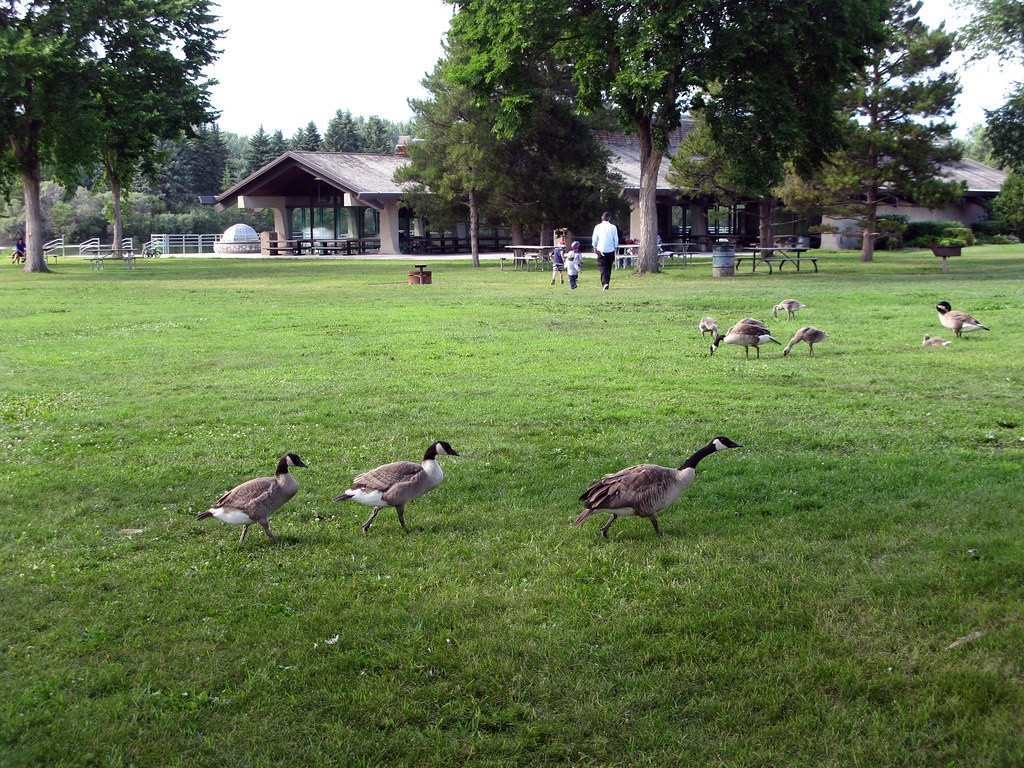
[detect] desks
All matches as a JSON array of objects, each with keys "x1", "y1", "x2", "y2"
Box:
[
  {"x1": 741, "y1": 247, "x2": 807, "y2": 271},
  {"x1": 505, "y1": 243, "x2": 559, "y2": 270},
  {"x1": 14, "y1": 245, "x2": 56, "y2": 264},
  {"x1": 351, "y1": 239, "x2": 380, "y2": 253},
  {"x1": 267, "y1": 239, "x2": 314, "y2": 255},
  {"x1": 757, "y1": 233, "x2": 798, "y2": 247},
  {"x1": 415, "y1": 237, "x2": 458, "y2": 255},
  {"x1": 706, "y1": 233, "x2": 742, "y2": 249},
  {"x1": 618, "y1": 242, "x2": 696, "y2": 268},
  {"x1": 85, "y1": 248, "x2": 140, "y2": 270},
  {"x1": 316, "y1": 239, "x2": 357, "y2": 254}
]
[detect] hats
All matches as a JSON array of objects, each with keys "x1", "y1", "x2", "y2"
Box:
[
  {"x1": 559, "y1": 242, "x2": 567, "y2": 247},
  {"x1": 567, "y1": 251, "x2": 574, "y2": 258}
]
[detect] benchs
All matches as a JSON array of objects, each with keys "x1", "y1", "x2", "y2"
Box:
[
  {"x1": 268, "y1": 243, "x2": 497, "y2": 255},
  {"x1": 696, "y1": 241, "x2": 737, "y2": 250},
  {"x1": 760, "y1": 258, "x2": 820, "y2": 274},
  {"x1": 614, "y1": 251, "x2": 700, "y2": 272},
  {"x1": 83, "y1": 256, "x2": 144, "y2": 271},
  {"x1": 7, "y1": 254, "x2": 59, "y2": 265},
  {"x1": 731, "y1": 256, "x2": 770, "y2": 271},
  {"x1": 499, "y1": 255, "x2": 553, "y2": 272},
  {"x1": 750, "y1": 241, "x2": 803, "y2": 252}
]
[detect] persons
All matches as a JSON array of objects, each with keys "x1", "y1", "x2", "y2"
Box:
[
  {"x1": 12, "y1": 236, "x2": 26, "y2": 264},
  {"x1": 591, "y1": 212, "x2": 619, "y2": 289},
  {"x1": 617, "y1": 229, "x2": 631, "y2": 267},
  {"x1": 657, "y1": 235, "x2": 663, "y2": 254},
  {"x1": 549, "y1": 241, "x2": 583, "y2": 289}
]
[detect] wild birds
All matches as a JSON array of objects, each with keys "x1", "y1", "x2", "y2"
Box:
[
  {"x1": 331, "y1": 441, "x2": 460, "y2": 538},
  {"x1": 783, "y1": 326, "x2": 829, "y2": 358},
  {"x1": 573, "y1": 436, "x2": 744, "y2": 541},
  {"x1": 710, "y1": 317, "x2": 781, "y2": 359},
  {"x1": 922, "y1": 334, "x2": 953, "y2": 347},
  {"x1": 773, "y1": 299, "x2": 807, "y2": 322},
  {"x1": 699, "y1": 317, "x2": 718, "y2": 342},
  {"x1": 936, "y1": 301, "x2": 989, "y2": 339},
  {"x1": 197, "y1": 453, "x2": 309, "y2": 554}
]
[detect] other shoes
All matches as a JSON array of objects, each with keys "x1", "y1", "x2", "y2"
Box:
[{"x1": 603, "y1": 284, "x2": 608, "y2": 290}]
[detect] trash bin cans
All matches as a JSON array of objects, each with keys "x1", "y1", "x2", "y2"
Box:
[
  {"x1": 797, "y1": 233, "x2": 811, "y2": 248},
  {"x1": 553, "y1": 222, "x2": 573, "y2": 253},
  {"x1": 318, "y1": 242, "x2": 327, "y2": 255},
  {"x1": 672, "y1": 234, "x2": 690, "y2": 252}
]
